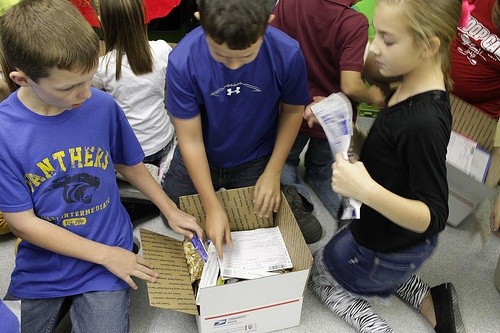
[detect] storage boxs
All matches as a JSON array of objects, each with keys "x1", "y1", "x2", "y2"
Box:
[
  {"x1": 445, "y1": 93, "x2": 500, "y2": 228},
  {"x1": 139, "y1": 187, "x2": 313, "y2": 333}
]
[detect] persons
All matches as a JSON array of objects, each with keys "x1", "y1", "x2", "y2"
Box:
[
  {"x1": 305, "y1": 0, "x2": 468, "y2": 333},
  {"x1": 0, "y1": 0, "x2": 500, "y2": 333}
]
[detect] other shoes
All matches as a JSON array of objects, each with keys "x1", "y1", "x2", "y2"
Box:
[
  {"x1": 338, "y1": 207, "x2": 351, "y2": 231},
  {"x1": 283, "y1": 186, "x2": 323, "y2": 244},
  {"x1": 431, "y1": 282, "x2": 466, "y2": 333}
]
